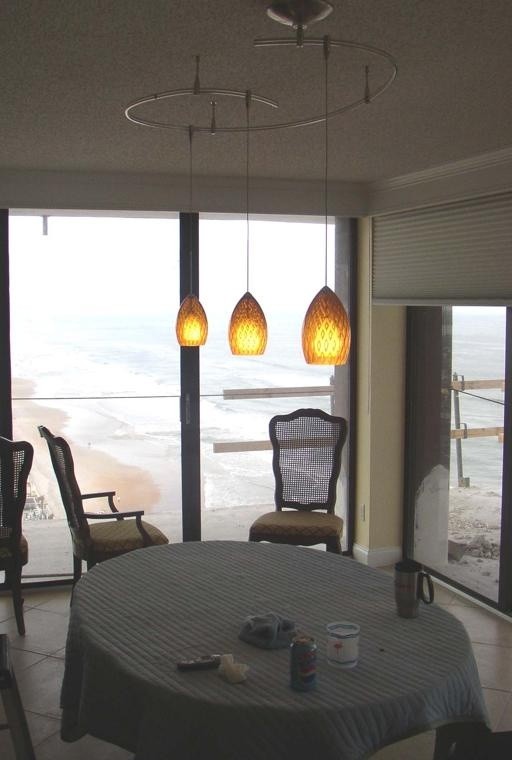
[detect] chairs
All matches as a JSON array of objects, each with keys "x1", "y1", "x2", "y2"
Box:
[
  {"x1": 450, "y1": 729, "x2": 512, "y2": 760},
  {"x1": 38, "y1": 424, "x2": 170, "y2": 605},
  {"x1": 1, "y1": 437, "x2": 30, "y2": 639},
  {"x1": 249, "y1": 409, "x2": 348, "y2": 554},
  {"x1": 0, "y1": 635, "x2": 36, "y2": 760}
]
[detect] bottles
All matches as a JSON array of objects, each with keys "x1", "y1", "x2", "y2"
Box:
[{"x1": 393, "y1": 559, "x2": 436, "y2": 619}]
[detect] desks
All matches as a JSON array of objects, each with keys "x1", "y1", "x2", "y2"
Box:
[{"x1": 58, "y1": 542, "x2": 488, "y2": 758}]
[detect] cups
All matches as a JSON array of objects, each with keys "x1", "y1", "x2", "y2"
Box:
[{"x1": 325, "y1": 620, "x2": 362, "y2": 670}]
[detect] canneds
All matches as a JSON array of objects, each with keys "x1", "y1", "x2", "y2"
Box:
[{"x1": 289, "y1": 635, "x2": 318, "y2": 692}]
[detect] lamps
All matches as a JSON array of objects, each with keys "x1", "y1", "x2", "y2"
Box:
[
  {"x1": 301, "y1": 42, "x2": 351, "y2": 367},
  {"x1": 229, "y1": 88, "x2": 267, "y2": 353},
  {"x1": 176, "y1": 124, "x2": 210, "y2": 347}
]
